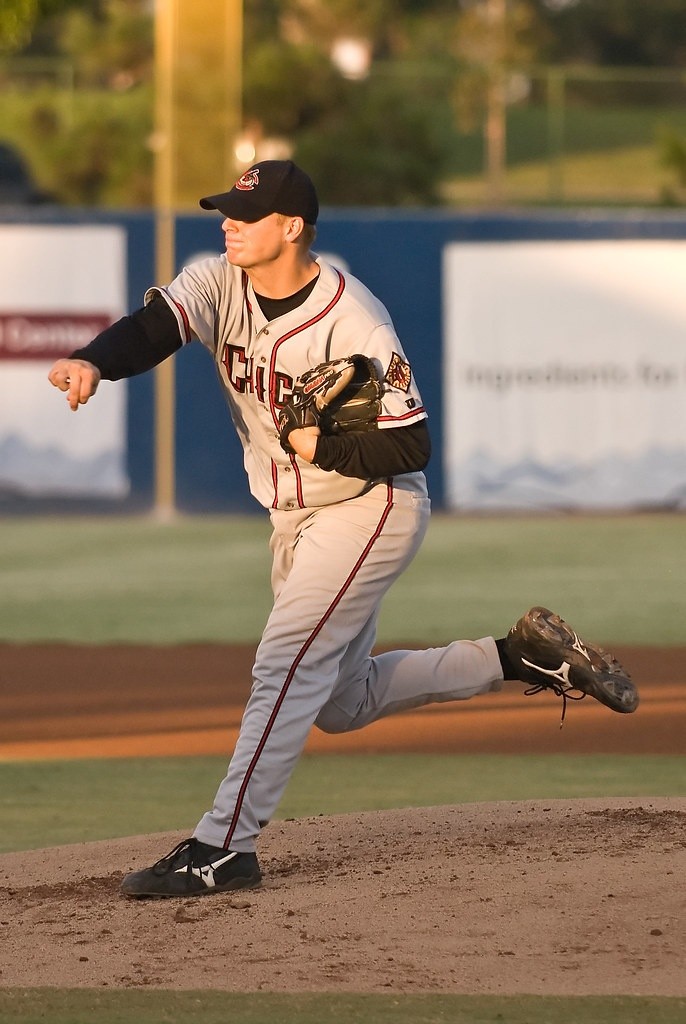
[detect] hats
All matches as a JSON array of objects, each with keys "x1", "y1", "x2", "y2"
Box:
[{"x1": 199, "y1": 160, "x2": 319, "y2": 225}]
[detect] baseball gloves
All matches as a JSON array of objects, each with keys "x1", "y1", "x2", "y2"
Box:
[{"x1": 272, "y1": 351, "x2": 389, "y2": 464}]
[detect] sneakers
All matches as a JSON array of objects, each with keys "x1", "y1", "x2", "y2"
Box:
[
  {"x1": 502, "y1": 607, "x2": 639, "y2": 729},
  {"x1": 120, "y1": 837, "x2": 262, "y2": 896}
]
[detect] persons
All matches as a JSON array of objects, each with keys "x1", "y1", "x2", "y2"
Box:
[{"x1": 48, "y1": 161, "x2": 639, "y2": 900}]
